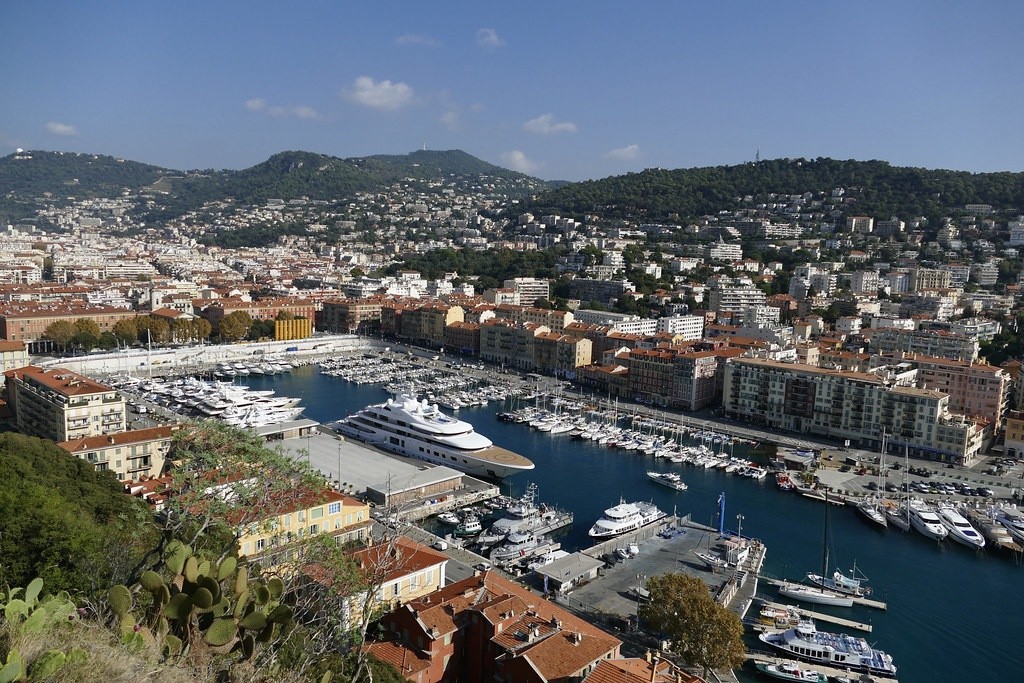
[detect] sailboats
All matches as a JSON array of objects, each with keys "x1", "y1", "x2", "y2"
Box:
[
  {"x1": 778, "y1": 486, "x2": 854, "y2": 608},
  {"x1": 856, "y1": 424, "x2": 890, "y2": 528},
  {"x1": 873, "y1": 439, "x2": 916, "y2": 532}
]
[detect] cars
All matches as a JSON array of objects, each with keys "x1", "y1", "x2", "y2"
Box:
[
  {"x1": 841, "y1": 465, "x2": 851, "y2": 473},
  {"x1": 893, "y1": 456, "x2": 1017, "y2": 500},
  {"x1": 868, "y1": 481, "x2": 878, "y2": 492},
  {"x1": 828, "y1": 455, "x2": 834, "y2": 461},
  {"x1": 884, "y1": 479, "x2": 898, "y2": 493},
  {"x1": 858, "y1": 468, "x2": 868, "y2": 475}
]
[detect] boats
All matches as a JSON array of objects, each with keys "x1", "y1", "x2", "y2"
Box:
[
  {"x1": 958, "y1": 506, "x2": 1023, "y2": 555},
  {"x1": 755, "y1": 660, "x2": 819, "y2": 683},
  {"x1": 832, "y1": 571, "x2": 861, "y2": 587},
  {"x1": 760, "y1": 622, "x2": 898, "y2": 676},
  {"x1": 758, "y1": 608, "x2": 813, "y2": 628},
  {"x1": 929, "y1": 501, "x2": 986, "y2": 551},
  {"x1": 902, "y1": 496, "x2": 949, "y2": 541},
  {"x1": 976, "y1": 502, "x2": 1024, "y2": 542},
  {"x1": 96, "y1": 346, "x2": 787, "y2": 577},
  {"x1": 807, "y1": 572, "x2": 871, "y2": 599}
]
